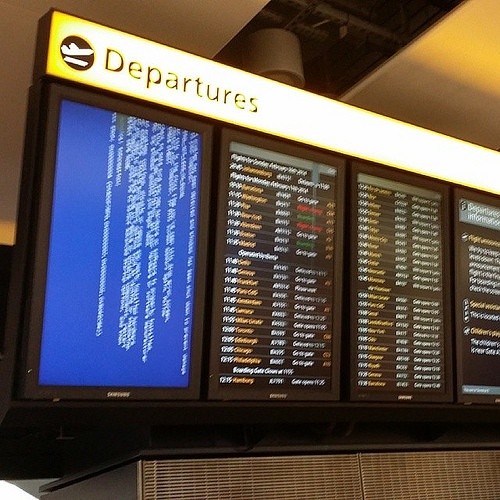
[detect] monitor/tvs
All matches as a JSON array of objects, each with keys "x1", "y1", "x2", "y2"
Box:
[
  {"x1": 210, "y1": 125, "x2": 347, "y2": 402},
  {"x1": 17, "y1": 83, "x2": 214, "y2": 402},
  {"x1": 351, "y1": 166, "x2": 454, "y2": 406},
  {"x1": 452, "y1": 185, "x2": 500, "y2": 404}
]
